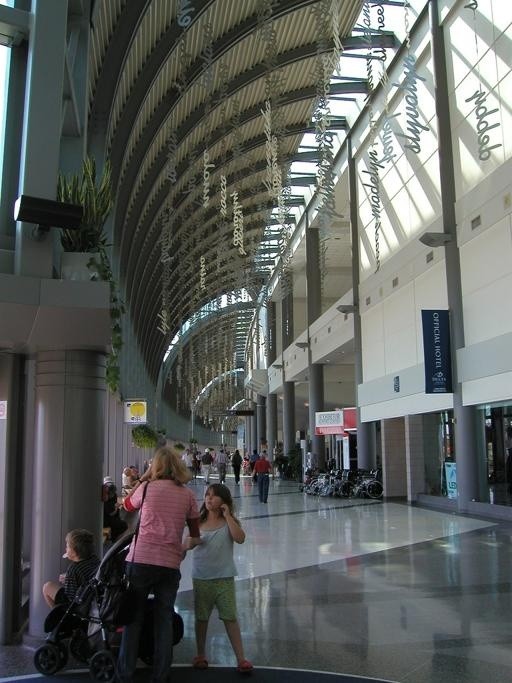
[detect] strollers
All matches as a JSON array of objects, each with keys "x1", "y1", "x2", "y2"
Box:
[{"x1": 33, "y1": 531, "x2": 154, "y2": 682}]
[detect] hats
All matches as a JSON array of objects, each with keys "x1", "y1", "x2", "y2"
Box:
[{"x1": 103, "y1": 476, "x2": 116, "y2": 484}]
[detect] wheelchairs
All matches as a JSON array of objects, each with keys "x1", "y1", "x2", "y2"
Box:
[{"x1": 305, "y1": 469, "x2": 383, "y2": 500}]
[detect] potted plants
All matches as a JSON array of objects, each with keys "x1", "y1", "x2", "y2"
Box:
[{"x1": 52, "y1": 154, "x2": 120, "y2": 283}]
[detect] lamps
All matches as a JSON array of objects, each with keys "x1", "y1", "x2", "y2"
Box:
[
  {"x1": 271, "y1": 364, "x2": 282, "y2": 369},
  {"x1": 295, "y1": 341, "x2": 308, "y2": 348},
  {"x1": 418, "y1": 229, "x2": 450, "y2": 248},
  {"x1": 336, "y1": 304, "x2": 354, "y2": 313},
  {"x1": 13, "y1": 193, "x2": 85, "y2": 243}
]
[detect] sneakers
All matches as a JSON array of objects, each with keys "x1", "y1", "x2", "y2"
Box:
[
  {"x1": 192, "y1": 656, "x2": 209, "y2": 669},
  {"x1": 234, "y1": 659, "x2": 255, "y2": 673}
]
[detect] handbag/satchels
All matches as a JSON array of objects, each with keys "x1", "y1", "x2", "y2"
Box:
[{"x1": 113, "y1": 588, "x2": 135, "y2": 626}]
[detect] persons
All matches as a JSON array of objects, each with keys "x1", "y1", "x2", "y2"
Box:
[
  {"x1": 113, "y1": 447, "x2": 203, "y2": 683},
  {"x1": 121, "y1": 447, "x2": 273, "y2": 504},
  {"x1": 43, "y1": 528, "x2": 105, "y2": 641},
  {"x1": 182, "y1": 483, "x2": 255, "y2": 674}
]
